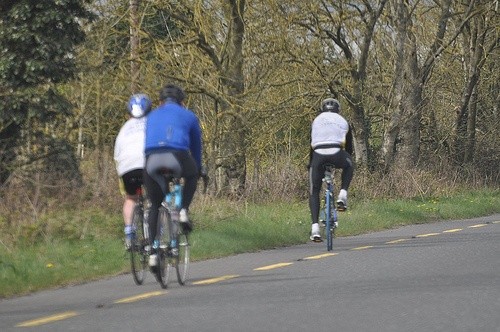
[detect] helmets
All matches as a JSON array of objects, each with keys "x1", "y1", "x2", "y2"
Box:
[
  {"x1": 320, "y1": 98, "x2": 339, "y2": 112},
  {"x1": 127, "y1": 93, "x2": 151, "y2": 118},
  {"x1": 159, "y1": 84, "x2": 184, "y2": 101}
]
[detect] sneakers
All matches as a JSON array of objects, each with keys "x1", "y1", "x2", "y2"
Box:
[
  {"x1": 310, "y1": 230, "x2": 321, "y2": 241},
  {"x1": 336, "y1": 196, "x2": 347, "y2": 212}
]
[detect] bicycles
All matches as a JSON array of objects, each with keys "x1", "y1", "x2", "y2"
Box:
[
  {"x1": 307, "y1": 152, "x2": 354, "y2": 251},
  {"x1": 152, "y1": 172, "x2": 210, "y2": 289},
  {"x1": 122, "y1": 167, "x2": 171, "y2": 285}
]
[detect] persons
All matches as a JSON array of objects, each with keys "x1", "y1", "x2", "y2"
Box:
[
  {"x1": 111, "y1": 93, "x2": 152, "y2": 250},
  {"x1": 143, "y1": 83, "x2": 203, "y2": 270},
  {"x1": 305, "y1": 99, "x2": 355, "y2": 241}
]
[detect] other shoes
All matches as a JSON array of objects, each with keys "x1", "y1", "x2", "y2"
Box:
[
  {"x1": 148, "y1": 254, "x2": 158, "y2": 266},
  {"x1": 125, "y1": 239, "x2": 136, "y2": 251},
  {"x1": 179, "y1": 212, "x2": 192, "y2": 232}
]
